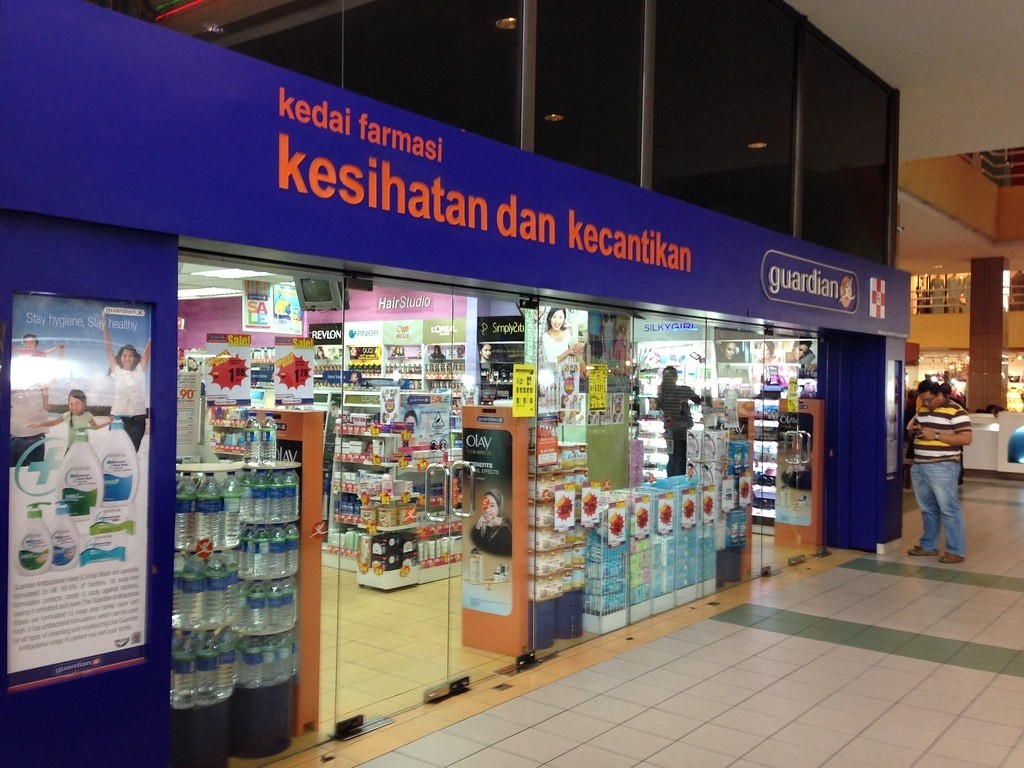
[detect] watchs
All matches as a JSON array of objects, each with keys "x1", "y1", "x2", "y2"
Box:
[{"x1": 934, "y1": 432, "x2": 940, "y2": 441}]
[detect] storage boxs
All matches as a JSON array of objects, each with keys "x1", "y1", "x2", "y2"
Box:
[
  {"x1": 371, "y1": 534, "x2": 397, "y2": 556},
  {"x1": 529, "y1": 501, "x2": 555, "y2": 529},
  {"x1": 358, "y1": 534, "x2": 378, "y2": 553},
  {"x1": 528, "y1": 571, "x2": 563, "y2": 603},
  {"x1": 529, "y1": 548, "x2": 565, "y2": 577},
  {"x1": 528, "y1": 471, "x2": 564, "y2": 502},
  {"x1": 529, "y1": 526, "x2": 566, "y2": 552},
  {"x1": 399, "y1": 550, "x2": 418, "y2": 569},
  {"x1": 371, "y1": 554, "x2": 400, "y2": 571},
  {"x1": 358, "y1": 553, "x2": 371, "y2": 569},
  {"x1": 394, "y1": 532, "x2": 417, "y2": 553}
]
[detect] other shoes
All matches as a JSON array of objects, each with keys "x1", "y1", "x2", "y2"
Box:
[
  {"x1": 940, "y1": 551, "x2": 964, "y2": 563},
  {"x1": 907, "y1": 545, "x2": 940, "y2": 556}
]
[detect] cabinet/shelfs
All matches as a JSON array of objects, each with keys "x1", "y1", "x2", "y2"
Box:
[
  {"x1": 314, "y1": 361, "x2": 466, "y2": 391},
  {"x1": 355, "y1": 460, "x2": 462, "y2": 591},
  {"x1": 735, "y1": 397, "x2": 777, "y2": 536},
  {"x1": 321, "y1": 432, "x2": 403, "y2": 573}
]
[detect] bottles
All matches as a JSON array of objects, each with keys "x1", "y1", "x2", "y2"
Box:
[
  {"x1": 400, "y1": 347, "x2": 404, "y2": 356},
  {"x1": 261, "y1": 413, "x2": 277, "y2": 464},
  {"x1": 387, "y1": 364, "x2": 422, "y2": 374},
  {"x1": 724, "y1": 383, "x2": 752, "y2": 398},
  {"x1": 396, "y1": 346, "x2": 400, "y2": 356},
  {"x1": 170, "y1": 468, "x2": 299, "y2": 708},
  {"x1": 244, "y1": 412, "x2": 260, "y2": 465}
]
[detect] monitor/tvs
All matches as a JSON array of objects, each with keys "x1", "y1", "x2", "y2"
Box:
[{"x1": 292, "y1": 276, "x2": 343, "y2": 310}]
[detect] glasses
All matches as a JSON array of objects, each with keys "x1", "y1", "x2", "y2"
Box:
[{"x1": 919, "y1": 394, "x2": 936, "y2": 404}]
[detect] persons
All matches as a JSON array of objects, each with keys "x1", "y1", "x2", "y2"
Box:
[
  {"x1": 10, "y1": 308, "x2": 627, "y2": 560},
  {"x1": 716, "y1": 340, "x2": 815, "y2": 400},
  {"x1": 905, "y1": 379, "x2": 975, "y2": 564},
  {"x1": 657, "y1": 366, "x2": 703, "y2": 477}
]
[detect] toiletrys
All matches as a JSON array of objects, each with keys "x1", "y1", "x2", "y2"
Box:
[
  {"x1": 329, "y1": 408, "x2": 463, "y2": 577},
  {"x1": 15, "y1": 415, "x2": 140, "y2": 579}
]
